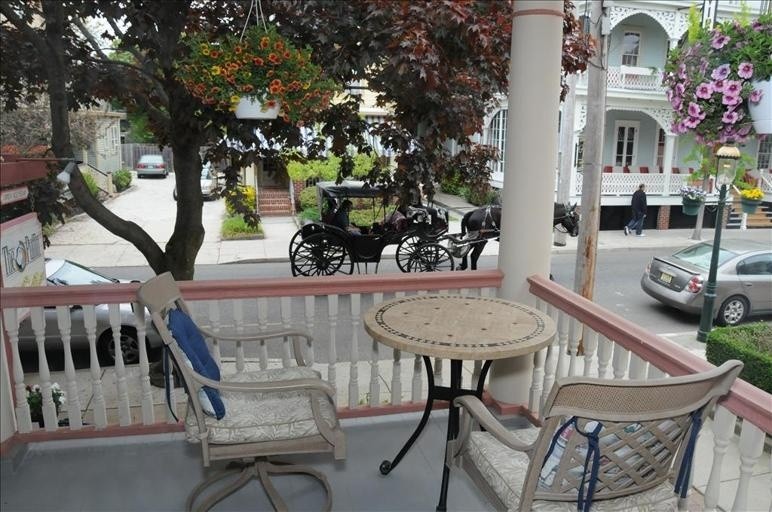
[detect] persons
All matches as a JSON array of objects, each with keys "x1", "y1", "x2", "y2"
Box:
[
  {"x1": 409, "y1": 179, "x2": 437, "y2": 224},
  {"x1": 624, "y1": 183, "x2": 647, "y2": 237},
  {"x1": 333, "y1": 199, "x2": 362, "y2": 235},
  {"x1": 324, "y1": 199, "x2": 338, "y2": 224}
]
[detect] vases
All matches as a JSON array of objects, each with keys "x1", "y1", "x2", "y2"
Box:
[
  {"x1": 741, "y1": 197, "x2": 762, "y2": 214},
  {"x1": 747, "y1": 78, "x2": 772, "y2": 134},
  {"x1": 682, "y1": 196, "x2": 704, "y2": 215},
  {"x1": 232, "y1": 86, "x2": 282, "y2": 120}
]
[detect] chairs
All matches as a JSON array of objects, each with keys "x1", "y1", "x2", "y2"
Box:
[
  {"x1": 605, "y1": 165, "x2": 694, "y2": 175},
  {"x1": 136, "y1": 270, "x2": 349, "y2": 512},
  {"x1": 444, "y1": 358, "x2": 745, "y2": 510}
]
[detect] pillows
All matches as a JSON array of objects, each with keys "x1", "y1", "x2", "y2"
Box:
[
  {"x1": 166, "y1": 309, "x2": 225, "y2": 421},
  {"x1": 532, "y1": 415, "x2": 690, "y2": 494}
]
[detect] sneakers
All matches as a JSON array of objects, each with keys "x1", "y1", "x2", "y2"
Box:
[{"x1": 624, "y1": 225, "x2": 645, "y2": 237}]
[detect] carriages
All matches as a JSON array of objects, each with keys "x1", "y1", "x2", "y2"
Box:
[{"x1": 287, "y1": 172, "x2": 580, "y2": 273}]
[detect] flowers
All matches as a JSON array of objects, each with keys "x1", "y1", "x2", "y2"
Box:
[
  {"x1": 740, "y1": 188, "x2": 764, "y2": 199},
  {"x1": 663, "y1": 4, "x2": 771, "y2": 145},
  {"x1": 173, "y1": 25, "x2": 338, "y2": 130},
  {"x1": 680, "y1": 185, "x2": 706, "y2": 200}
]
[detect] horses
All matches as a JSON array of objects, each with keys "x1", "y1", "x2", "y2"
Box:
[{"x1": 456, "y1": 202, "x2": 580, "y2": 270}]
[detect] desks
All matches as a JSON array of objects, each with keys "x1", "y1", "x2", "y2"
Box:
[{"x1": 362, "y1": 293, "x2": 556, "y2": 511}]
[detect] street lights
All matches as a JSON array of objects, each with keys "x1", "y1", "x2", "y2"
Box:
[{"x1": 694, "y1": 136, "x2": 743, "y2": 343}]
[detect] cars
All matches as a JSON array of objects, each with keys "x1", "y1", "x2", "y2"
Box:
[
  {"x1": 172, "y1": 167, "x2": 219, "y2": 201},
  {"x1": 13, "y1": 255, "x2": 165, "y2": 366},
  {"x1": 135, "y1": 154, "x2": 169, "y2": 178},
  {"x1": 639, "y1": 237, "x2": 772, "y2": 330}
]
